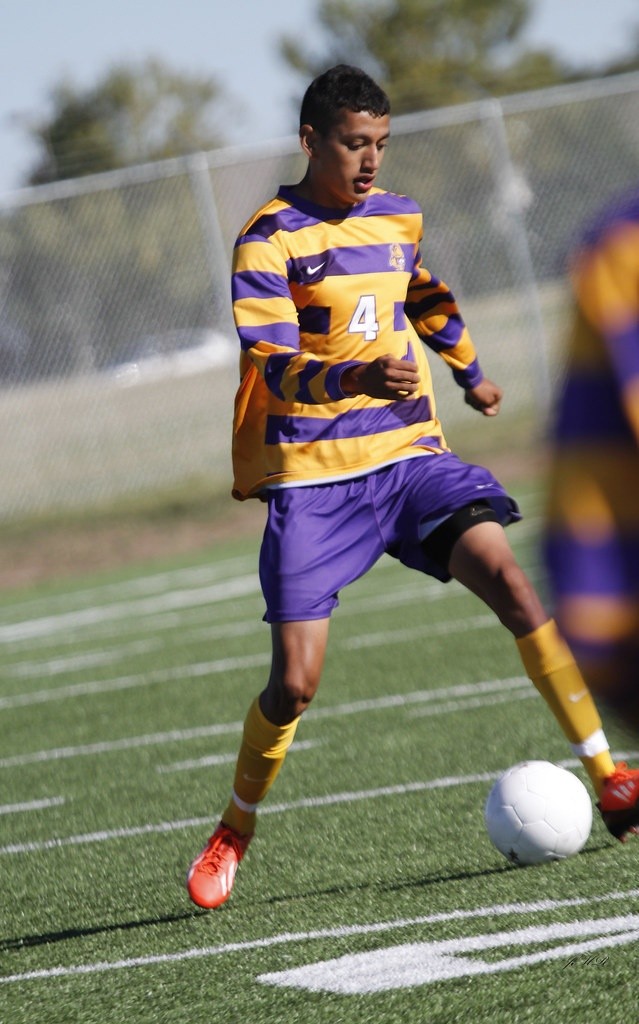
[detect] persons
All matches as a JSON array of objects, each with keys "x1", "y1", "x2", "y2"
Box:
[
  {"x1": 540, "y1": 188, "x2": 639, "y2": 736},
  {"x1": 184, "y1": 62, "x2": 639, "y2": 910}
]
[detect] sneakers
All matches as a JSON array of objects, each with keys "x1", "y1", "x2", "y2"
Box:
[
  {"x1": 187, "y1": 820, "x2": 254, "y2": 908},
  {"x1": 594, "y1": 762, "x2": 639, "y2": 843}
]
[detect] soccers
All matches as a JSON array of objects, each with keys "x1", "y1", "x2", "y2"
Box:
[{"x1": 485, "y1": 760, "x2": 592, "y2": 866}]
